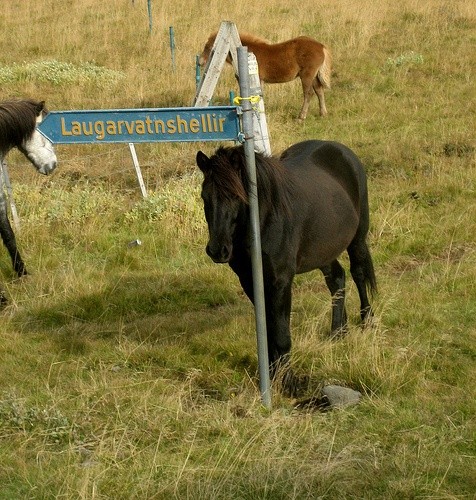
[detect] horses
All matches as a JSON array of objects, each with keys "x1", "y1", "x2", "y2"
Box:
[
  {"x1": 0, "y1": 100, "x2": 57, "y2": 310},
  {"x1": 196, "y1": 140, "x2": 378, "y2": 398},
  {"x1": 197, "y1": 31, "x2": 330, "y2": 124}
]
